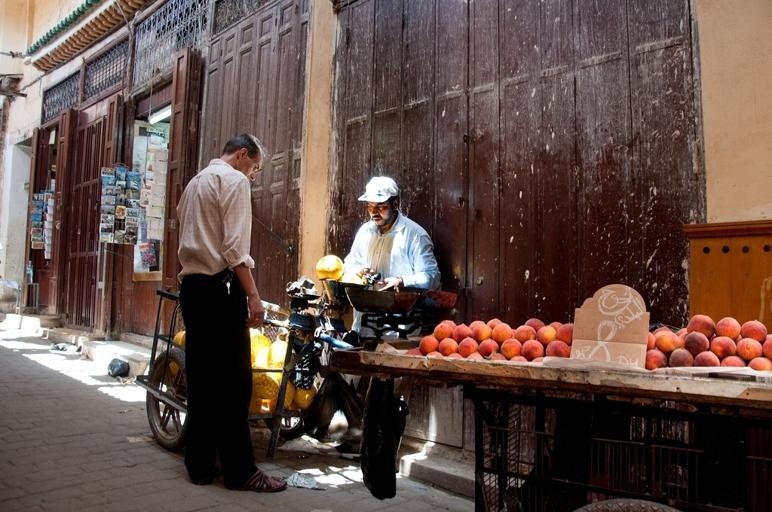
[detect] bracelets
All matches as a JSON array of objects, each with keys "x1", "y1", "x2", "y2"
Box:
[
  {"x1": 343, "y1": 285, "x2": 420, "y2": 314},
  {"x1": 322, "y1": 278, "x2": 367, "y2": 308}
]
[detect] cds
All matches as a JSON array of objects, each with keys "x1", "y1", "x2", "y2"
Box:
[{"x1": 226, "y1": 282, "x2": 231, "y2": 294}]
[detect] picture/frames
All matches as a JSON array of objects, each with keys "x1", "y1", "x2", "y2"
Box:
[{"x1": 358, "y1": 349, "x2": 772, "y2": 512}]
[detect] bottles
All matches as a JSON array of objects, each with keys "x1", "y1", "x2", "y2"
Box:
[{"x1": 357, "y1": 176, "x2": 399, "y2": 203}]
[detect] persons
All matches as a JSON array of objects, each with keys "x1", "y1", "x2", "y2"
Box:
[
  {"x1": 176, "y1": 131, "x2": 287, "y2": 493},
  {"x1": 338, "y1": 176, "x2": 442, "y2": 444}
]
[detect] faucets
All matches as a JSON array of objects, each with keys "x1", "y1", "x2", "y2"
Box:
[{"x1": 238, "y1": 469, "x2": 288, "y2": 492}]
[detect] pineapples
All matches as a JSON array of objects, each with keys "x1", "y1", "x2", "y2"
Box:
[
  {"x1": 419, "y1": 317, "x2": 573, "y2": 361},
  {"x1": 646, "y1": 314, "x2": 772, "y2": 371}
]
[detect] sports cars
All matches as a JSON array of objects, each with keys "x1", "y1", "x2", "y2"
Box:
[{"x1": 128, "y1": 283, "x2": 355, "y2": 461}]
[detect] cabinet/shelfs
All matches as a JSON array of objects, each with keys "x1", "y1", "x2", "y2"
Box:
[{"x1": 25, "y1": 260, "x2": 34, "y2": 284}]
